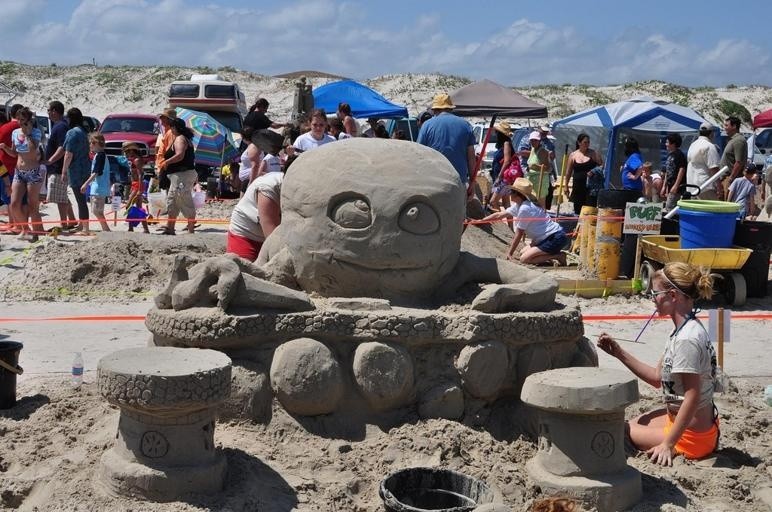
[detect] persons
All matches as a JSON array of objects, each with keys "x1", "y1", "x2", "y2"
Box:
[
  {"x1": 156, "y1": 118, "x2": 197, "y2": 234},
  {"x1": 1, "y1": 101, "x2": 110, "y2": 241},
  {"x1": 122, "y1": 142, "x2": 150, "y2": 233},
  {"x1": 227, "y1": 154, "x2": 297, "y2": 261},
  {"x1": 221, "y1": 99, "x2": 405, "y2": 200},
  {"x1": 416, "y1": 111, "x2": 431, "y2": 127},
  {"x1": 471, "y1": 117, "x2": 772, "y2": 267},
  {"x1": 150, "y1": 107, "x2": 177, "y2": 225},
  {"x1": 416, "y1": 94, "x2": 478, "y2": 199},
  {"x1": 597, "y1": 262, "x2": 720, "y2": 468}
]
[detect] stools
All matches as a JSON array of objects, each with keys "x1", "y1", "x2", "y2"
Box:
[
  {"x1": 520, "y1": 365, "x2": 643, "y2": 511},
  {"x1": 95, "y1": 347, "x2": 233, "y2": 500}
]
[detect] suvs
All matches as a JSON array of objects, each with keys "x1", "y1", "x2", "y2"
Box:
[
  {"x1": 509, "y1": 123, "x2": 578, "y2": 157},
  {"x1": 470, "y1": 120, "x2": 523, "y2": 168},
  {"x1": 86, "y1": 113, "x2": 162, "y2": 173}
]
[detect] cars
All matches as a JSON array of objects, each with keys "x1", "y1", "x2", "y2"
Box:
[
  {"x1": 0, "y1": 103, "x2": 15, "y2": 128},
  {"x1": 32, "y1": 110, "x2": 102, "y2": 140},
  {"x1": 748, "y1": 127, "x2": 771, "y2": 173}
]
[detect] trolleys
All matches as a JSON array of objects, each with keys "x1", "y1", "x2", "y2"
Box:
[{"x1": 636, "y1": 184, "x2": 754, "y2": 307}]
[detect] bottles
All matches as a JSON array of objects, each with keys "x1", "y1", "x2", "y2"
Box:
[{"x1": 71, "y1": 350, "x2": 85, "y2": 391}]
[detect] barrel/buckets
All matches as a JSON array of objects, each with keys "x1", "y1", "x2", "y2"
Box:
[
  {"x1": 676, "y1": 199, "x2": 740, "y2": 249},
  {"x1": 0, "y1": 341, "x2": 23, "y2": 410},
  {"x1": 378, "y1": 467, "x2": 495, "y2": 512},
  {"x1": 127, "y1": 206, "x2": 148, "y2": 227},
  {"x1": 148, "y1": 189, "x2": 168, "y2": 215},
  {"x1": 192, "y1": 191, "x2": 208, "y2": 209}
]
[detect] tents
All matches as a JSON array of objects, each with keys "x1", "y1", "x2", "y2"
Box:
[{"x1": 552, "y1": 99, "x2": 722, "y2": 190}]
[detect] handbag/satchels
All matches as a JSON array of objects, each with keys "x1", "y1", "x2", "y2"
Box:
[{"x1": 499, "y1": 154, "x2": 522, "y2": 184}]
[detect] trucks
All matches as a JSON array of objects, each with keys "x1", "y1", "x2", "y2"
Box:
[{"x1": 167, "y1": 71, "x2": 247, "y2": 140}]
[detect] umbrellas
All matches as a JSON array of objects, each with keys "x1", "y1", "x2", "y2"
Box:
[{"x1": 175, "y1": 106, "x2": 240, "y2": 166}]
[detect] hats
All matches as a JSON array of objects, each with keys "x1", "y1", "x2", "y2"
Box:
[
  {"x1": 493, "y1": 121, "x2": 512, "y2": 137},
  {"x1": 529, "y1": 132, "x2": 540, "y2": 140},
  {"x1": 431, "y1": 95, "x2": 456, "y2": 109},
  {"x1": 700, "y1": 122, "x2": 715, "y2": 131},
  {"x1": 157, "y1": 109, "x2": 176, "y2": 121},
  {"x1": 507, "y1": 177, "x2": 536, "y2": 202},
  {"x1": 122, "y1": 142, "x2": 138, "y2": 152},
  {"x1": 746, "y1": 164, "x2": 755, "y2": 174}
]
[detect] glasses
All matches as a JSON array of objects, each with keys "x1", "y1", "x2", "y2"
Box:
[{"x1": 651, "y1": 289, "x2": 669, "y2": 298}]
